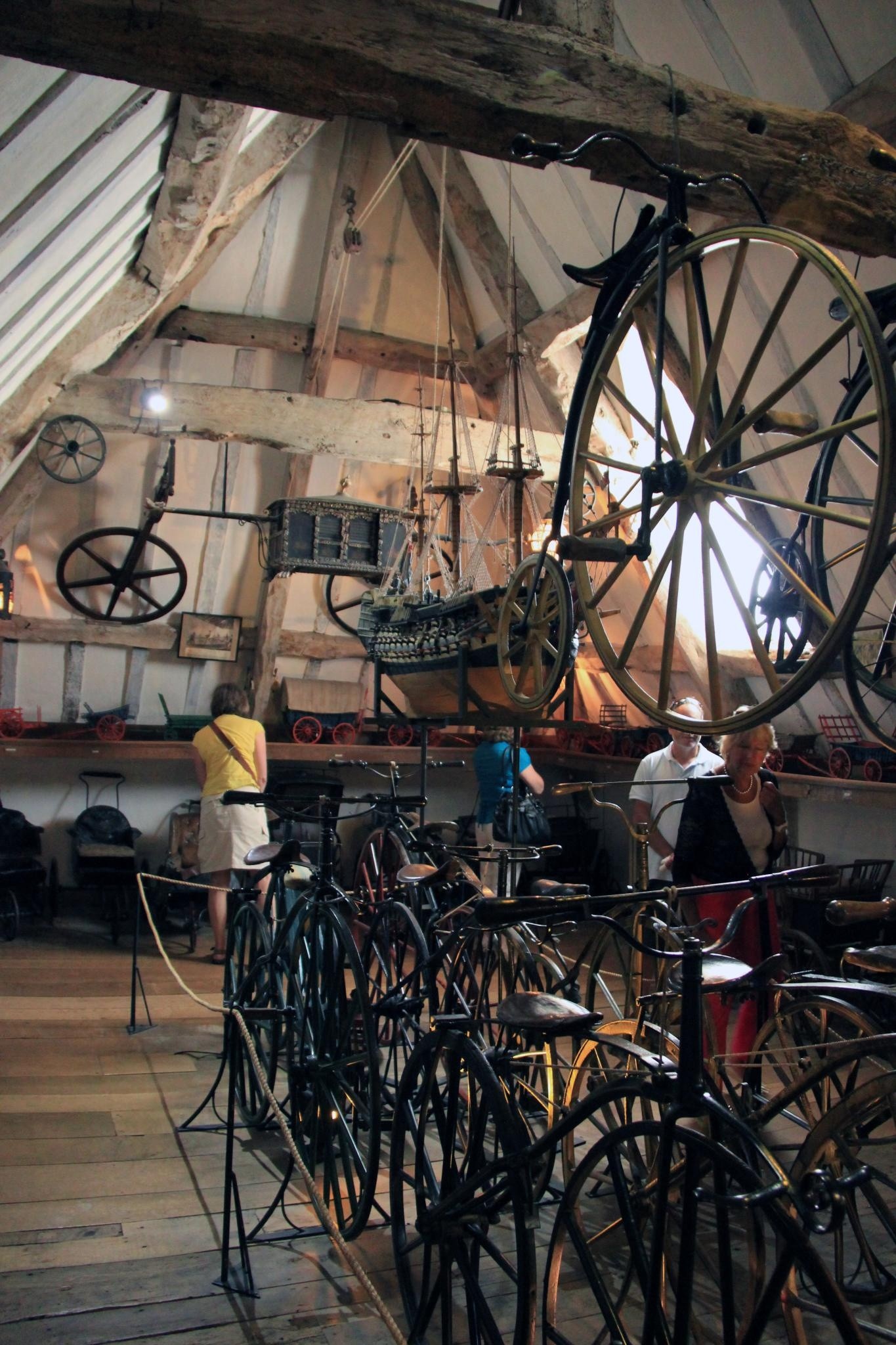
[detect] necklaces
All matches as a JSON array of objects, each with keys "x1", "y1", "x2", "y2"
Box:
[{"x1": 722, "y1": 762, "x2": 754, "y2": 795}]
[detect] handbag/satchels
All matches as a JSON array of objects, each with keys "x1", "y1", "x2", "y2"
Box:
[{"x1": 493, "y1": 745, "x2": 553, "y2": 844}]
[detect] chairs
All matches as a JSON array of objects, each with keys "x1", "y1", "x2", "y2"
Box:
[{"x1": 764, "y1": 843, "x2": 896, "y2": 997}]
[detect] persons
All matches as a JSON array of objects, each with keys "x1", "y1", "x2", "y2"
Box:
[
  {"x1": 627, "y1": 697, "x2": 726, "y2": 1025},
  {"x1": 472, "y1": 724, "x2": 545, "y2": 968},
  {"x1": 670, "y1": 703, "x2": 791, "y2": 1101},
  {"x1": 190, "y1": 680, "x2": 279, "y2": 967}
]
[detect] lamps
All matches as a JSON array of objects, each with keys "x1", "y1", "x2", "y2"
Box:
[{"x1": 139, "y1": 388, "x2": 167, "y2": 413}]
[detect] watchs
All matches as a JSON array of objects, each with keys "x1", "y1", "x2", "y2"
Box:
[{"x1": 773, "y1": 820, "x2": 788, "y2": 833}]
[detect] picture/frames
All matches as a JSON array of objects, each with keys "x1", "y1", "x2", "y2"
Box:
[{"x1": 178, "y1": 611, "x2": 242, "y2": 662}]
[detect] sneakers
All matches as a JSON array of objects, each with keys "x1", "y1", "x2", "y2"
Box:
[{"x1": 725, "y1": 1057, "x2": 771, "y2": 1096}]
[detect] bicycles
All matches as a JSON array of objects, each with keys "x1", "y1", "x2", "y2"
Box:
[{"x1": 149, "y1": 748, "x2": 896, "y2": 1344}]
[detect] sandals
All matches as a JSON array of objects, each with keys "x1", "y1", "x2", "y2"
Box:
[{"x1": 210, "y1": 946, "x2": 227, "y2": 964}]
